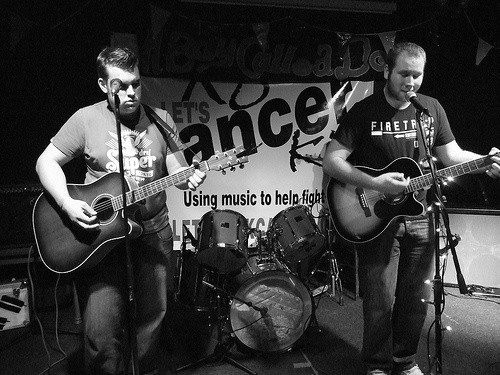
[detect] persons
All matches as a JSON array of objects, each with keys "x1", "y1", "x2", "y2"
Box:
[
  {"x1": 36, "y1": 47, "x2": 207, "y2": 375},
  {"x1": 323, "y1": 43, "x2": 500, "y2": 375}
]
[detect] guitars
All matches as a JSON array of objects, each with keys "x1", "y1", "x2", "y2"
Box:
[
  {"x1": 326, "y1": 151, "x2": 500, "y2": 244},
  {"x1": 31, "y1": 144, "x2": 249, "y2": 274}
]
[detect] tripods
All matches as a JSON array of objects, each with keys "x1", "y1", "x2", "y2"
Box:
[
  {"x1": 177, "y1": 282, "x2": 267, "y2": 375},
  {"x1": 307, "y1": 218, "x2": 344, "y2": 305}
]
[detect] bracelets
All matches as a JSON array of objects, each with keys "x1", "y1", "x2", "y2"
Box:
[{"x1": 60, "y1": 197, "x2": 71, "y2": 210}]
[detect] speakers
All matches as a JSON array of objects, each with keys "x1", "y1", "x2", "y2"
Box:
[{"x1": 0, "y1": 185, "x2": 43, "y2": 248}]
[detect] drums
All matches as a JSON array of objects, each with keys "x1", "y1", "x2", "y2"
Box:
[
  {"x1": 195, "y1": 209, "x2": 248, "y2": 274},
  {"x1": 225, "y1": 252, "x2": 316, "y2": 354},
  {"x1": 268, "y1": 204, "x2": 326, "y2": 264}
]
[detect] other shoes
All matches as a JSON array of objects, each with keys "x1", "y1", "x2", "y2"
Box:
[
  {"x1": 367, "y1": 368, "x2": 391, "y2": 375},
  {"x1": 393, "y1": 364, "x2": 424, "y2": 375}
]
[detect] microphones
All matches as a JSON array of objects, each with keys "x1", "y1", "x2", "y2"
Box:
[
  {"x1": 405, "y1": 91, "x2": 433, "y2": 118},
  {"x1": 110, "y1": 78, "x2": 122, "y2": 95}
]
